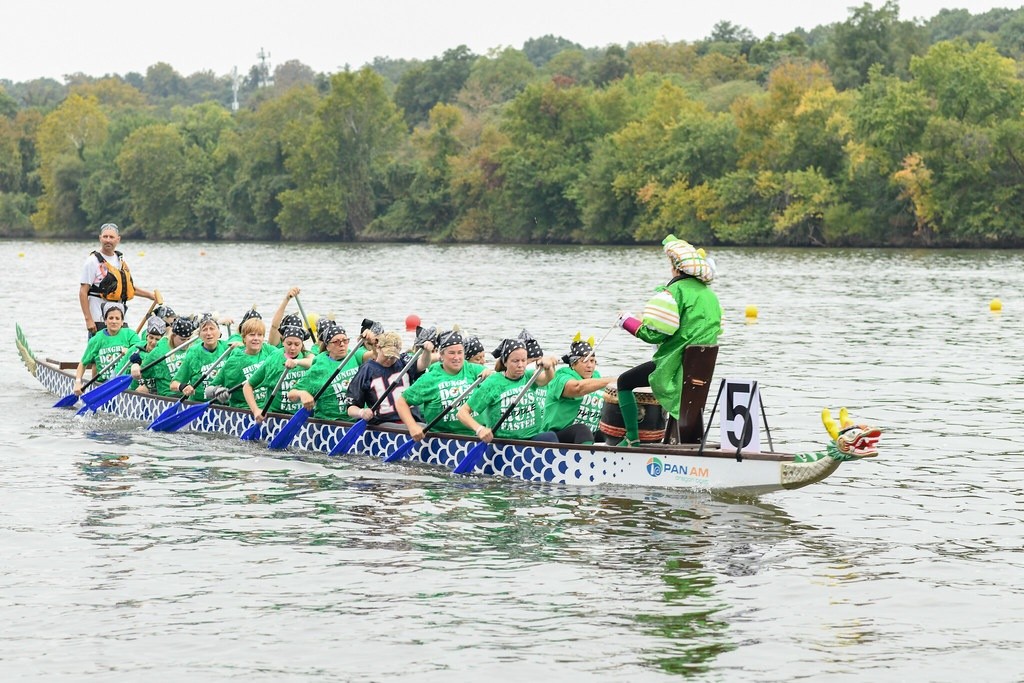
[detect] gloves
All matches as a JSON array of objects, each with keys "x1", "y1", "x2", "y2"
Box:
[{"x1": 215, "y1": 386, "x2": 232, "y2": 404}]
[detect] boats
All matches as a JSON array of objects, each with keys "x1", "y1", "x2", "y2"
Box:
[{"x1": 12, "y1": 321, "x2": 888, "y2": 497}]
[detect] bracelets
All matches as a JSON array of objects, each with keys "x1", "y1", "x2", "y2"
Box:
[
  {"x1": 476, "y1": 425, "x2": 484, "y2": 435},
  {"x1": 545, "y1": 367, "x2": 550, "y2": 370},
  {"x1": 179, "y1": 383, "x2": 188, "y2": 392}
]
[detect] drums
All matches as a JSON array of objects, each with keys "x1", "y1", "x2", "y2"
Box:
[{"x1": 597, "y1": 379, "x2": 667, "y2": 443}]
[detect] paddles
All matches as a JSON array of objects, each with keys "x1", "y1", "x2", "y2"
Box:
[
  {"x1": 382, "y1": 374, "x2": 482, "y2": 463},
  {"x1": 454, "y1": 364, "x2": 547, "y2": 474},
  {"x1": 294, "y1": 292, "x2": 316, "y2": 343},
  {"x1": 241, "y1": 364, "x2": 294, "y2": 440},
  {"x1": 151, "y1": 380, "x2": 248, "y2": 432},
  {"x1": 266, "y1": 334, "x2": 366, "y2": 450},
  {"x1": 75, "y1": 344, "x2": 143, "y2": 417},
  {"x1": 135, "y1": 286, "x2": 164, "y2": 336},
  {"x1": 80, "y1": 334, "x2": 199, "y2": 413},
  {"x1": 148, "y1": 344, "x2": 233, "y2": 430},
  {"x1": 53, "y1": 351, "x2": 125, "y2": 408},
  {"x1": 329, "y1": 344, "x2": 426, "y2": 455}
]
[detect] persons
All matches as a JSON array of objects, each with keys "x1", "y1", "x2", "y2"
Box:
[
  {"x1": 242, "y1": 324, "x2": 315, "y2": 424},
  {"x1": 204, "y1": 317, "x2": 278, "y2": 411},
  {"x1": 267, "y1": 287, "x2": 303, "y2": 349},
  {"x1": 344, "y1": 332, "x2": 435, "y2": 431},
  {"x1": 357, "y1": 318, "x2": 384, "y2": 350},
  {"x1": 542, "y1": 332, "x2": 618, "y2": 446},
  {"x1": 131, "y1": 317, "x2": 201, "y2": 399},
  {"x1": 395, "y1": 328, "x2": 492, "y2": 442},
  {"x1": 413, "y1": 324, "x2": 441, "y2": 372},
  {"x1": 79, "y1": 223, "x2": 155, "y2": 380},
  {"x1": 73, "y1": 302, "x2": 141, "y2": 396},
  {"x1": 463, "y1": 335, "x2": 486, "y2": 367},
  {"x1": 228, "y1": 305, "x2": 263, "y2": 345},
  {"x1": 494, "y1": 328, "x2": 558, "y2": 434},
  {"x1": 107, "y1": 315, "x2": 166, "y2": 394},
  {"x1": 612, "y1": 233, "x2": 721, "y2": 448},
  {"x1": 169, "y1": 313, "x2": 233, "y2": 406},
  {"x1": 152, "y1": 305, "x2": 235, "y2": 344},
  {"x1": 456, "y1": 337, "x2": 559, "y2": 443},
  {"x1": 287, "y1": 324, "x2": 378, "y2": 422},
  {"x1": 311, "y1": 319, "x2": 336, "y2": 355}
]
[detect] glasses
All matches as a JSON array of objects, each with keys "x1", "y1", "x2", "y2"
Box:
[
  {"x1": 101, "y1": 223, "x2": 119, "y2": 230},
  {"x1": 328, "y1": 338, "x2": 351, "y2": 347}
]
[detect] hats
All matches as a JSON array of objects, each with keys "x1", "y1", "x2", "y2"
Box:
[
  {"x1": 663, "y1": 233, "x2": 713, "y2": 283},
  {"x1": 377, "y1": 331, "x2": 402, "y2": 361}
]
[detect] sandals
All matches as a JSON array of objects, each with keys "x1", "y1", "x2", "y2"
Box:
[{"x1": 616, "y1": 436, "x2": 640, "y2": 447}]
